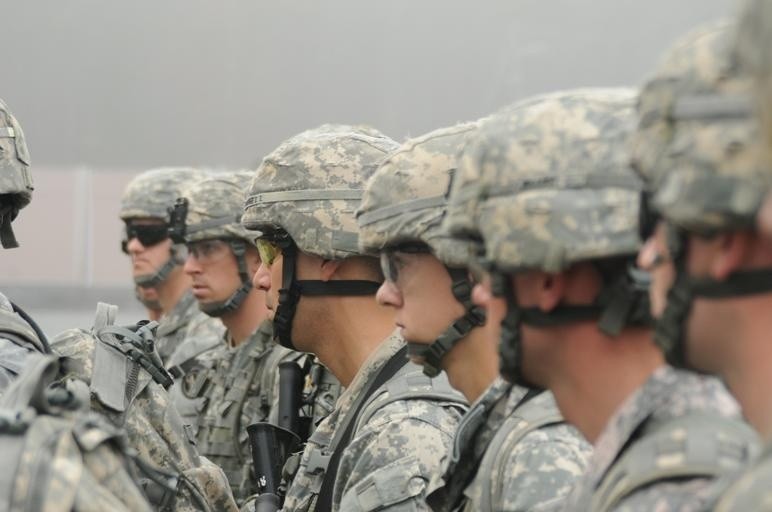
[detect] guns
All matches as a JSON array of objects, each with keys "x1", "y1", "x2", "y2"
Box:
[
  {"x1": 278, "y1": 362, "x2": 312, "y2": 443},
  {"x1": 246, "y1": 422, "x2": 301, "y2": 512}
]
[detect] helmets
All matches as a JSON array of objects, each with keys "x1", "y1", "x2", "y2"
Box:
[
  {"x1": 241, "y1": 121, "x2": 398, "y2": 260},
  {"x1": 358, "y1": 118, "x2": 475, "y2": 267},
  {"x1": 171, "y1": 169, "x2": 264, "y2": 244},
  {"x1": 630, "y1": 0, "x2": 772, "y2": 238},
  {"x1": 440, "y1": 86, "x2": 638, "y2": 275},
  {"x1": 0, "y1": 98, "x2": 35, "y2": 222},
  {"x1": 119, "y1": 168, "x2": 204, "y2": 223}
]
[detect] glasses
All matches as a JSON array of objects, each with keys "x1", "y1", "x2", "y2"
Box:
[
  {"x1": 122, "y1": 223, "x2": 172, "y2": 244},
  {"x1": 377, "y1": 245, "x2": 400, "y2": 284},
  {"x1": 180, "y1": 239, "x2": 222, "y2": 263},
  {"x1": 252, "y1": 230, "x2": 290, "y2": 262}
]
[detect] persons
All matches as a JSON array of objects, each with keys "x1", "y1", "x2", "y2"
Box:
[
  {"x1": 166, "y1": 169, "x2": 347, "y2": 511},
  {"x1": 353, "y1": 123, "x2": 595, "y2": 511},
  {"x1": 628, "y1": 1, "x2": 770, "y2": 511},
  {"x1": 241, "y1": 124, "x2": 471, "y2": 511},
  {"x1": 1, "y1": 99, "x2": 229, "y2": 512},
  {"x1": 439, "y1": 87, "x2": 772, "y2": 510}
]
